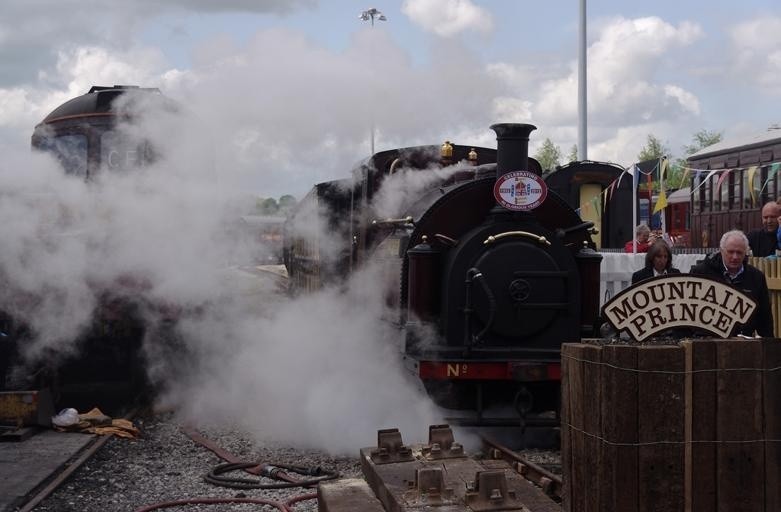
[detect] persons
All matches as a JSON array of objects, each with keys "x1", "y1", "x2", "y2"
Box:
[
  {"x1": 689, "y1": 228, "x2": 774, "y2": 338},
  {"x1": 632, "y1": 238, "x2": 680, "y2": 285},
  {"x1": 624, "y1": 224, "x2": 654, "y2": 252},
  {"x1": 744, "y1": 201, "x2": 781, "y2": 257},
  {"x1": 766, "y1": 196, "x2": 781, "y2": 259}
]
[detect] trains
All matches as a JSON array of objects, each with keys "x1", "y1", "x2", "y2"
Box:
[{"x1": 277, "y1": 123, "x2": 604, "y2": 425}]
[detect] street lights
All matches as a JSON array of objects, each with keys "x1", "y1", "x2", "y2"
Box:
[{"x1": 359, "y1": 7, "x2": 387, "y2": 155}]
